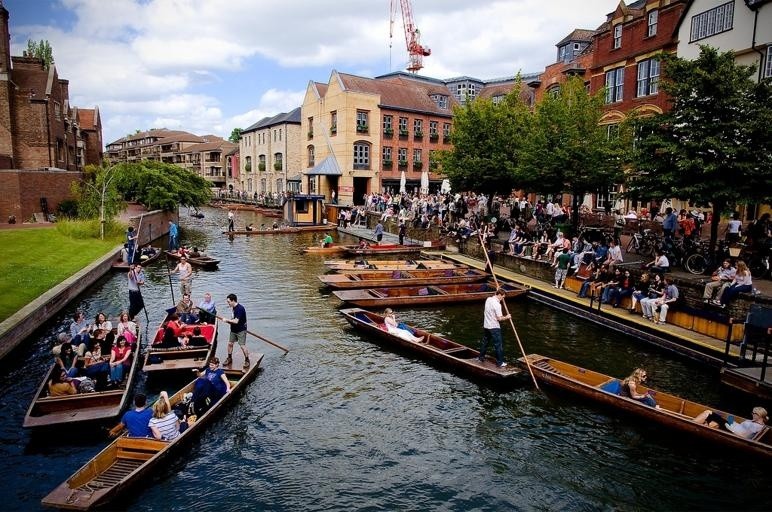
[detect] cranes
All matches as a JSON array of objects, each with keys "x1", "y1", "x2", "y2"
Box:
[{"x1": 386, "y1": 0, "x2": 432, "y2": 77}]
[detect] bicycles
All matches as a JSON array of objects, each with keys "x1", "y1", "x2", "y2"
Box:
[{"x1": 623, "y1": 225, "x2": 772, "y2": 276}]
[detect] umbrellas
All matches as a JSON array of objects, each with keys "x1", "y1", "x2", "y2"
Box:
[
  {"x1": 424, "y1": 171, "x2": 429, "y2": 194},
  {"x1": 420, "y1": 171, "x2": 424, "y2": 193},
  {"x1": 398, "y1": 170, "x2": 407, "y2": 194}
]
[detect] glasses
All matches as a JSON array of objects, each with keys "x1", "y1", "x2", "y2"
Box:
[
  {"x1": 641, "y1": 374, "x2": 647, "y2": 377},
  {"x1": 751, "y1": 411, "x2": 757, "y2": 415}
]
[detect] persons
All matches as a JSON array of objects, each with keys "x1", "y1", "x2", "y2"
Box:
[
  {"x1": 383, "y1": 307, "x2": 425, "y2": 343},
  {"x1": 478, "y1": 288, "x2": 512, "y2": 367},
  {"x1": 358, "y1": 187, "x2": 577, "y2": 289},
  {"x1": 254, "y1": 189, "x2": 305, "y2": 206},
  {"x1": 121, "y1": 227, "x2": 155, "y2": 264},
  {"x1": 691, "y1": 404, "x2": 770, "y2": 440},
  {"x1": 228, "y1": 209, "x2": 235, "y2": 232},
  {"x1": 168, "y1": 255, "x2": 193, "y2": 300},
  {"x1": 618, "y1": 367, "x2": 660, "y2": 409},
  {"x1": 168, "y1": 219, "x2": 179, "y2": 253},
  {"x1": 338, "y1": 205, "x2": 358, "y2": 230},
  {"x1": 45, "y1": 293, "x2": 230, "y2": 442},
  {"x1": 246, "y1": 223, "x2": 257, "y2": 230},
  {"x1": 218, "y1": 293, "x2": 251, "y2": 368},
  {"x1": 331, "y1": 188, "x2": 336, "y2": 204},
  {"x1": 177, "y1": 243, "x2": 208, "y2": 261},
  {"x1": 273, "y1": 222, "x2": 279, "y2": 230},
  {"x1": 574, "y1": 206, "x2": 771, "y2": 324},
  {"x1": 320, "y1": 232, "x2": 334, "y2": 249},
  {"x1": 126, "y1": 264, "x2": 147, "y2": 320},
  {"x1": 260, "y1": 224, "x2": 268, "y2": 231},
  {"x1": 220, "y1": 189, "x2": 250, "y2": 202}
]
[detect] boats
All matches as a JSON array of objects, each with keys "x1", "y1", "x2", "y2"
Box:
[
  {"x1": 190, "y1": 211, "x2": 204, "y2": 218},
  {"x1": 222, "y1": 223, "x2": 304, "y2": 235},
  {"x1": 164, "y1": 246, "x2": 220, "y2": 270},
  {"x1": 112, "y1": 243, "x2": 163, "y2": 271},
  {"x1": 208, "y1": 202, "x2": 283, "y2": 218}
]
